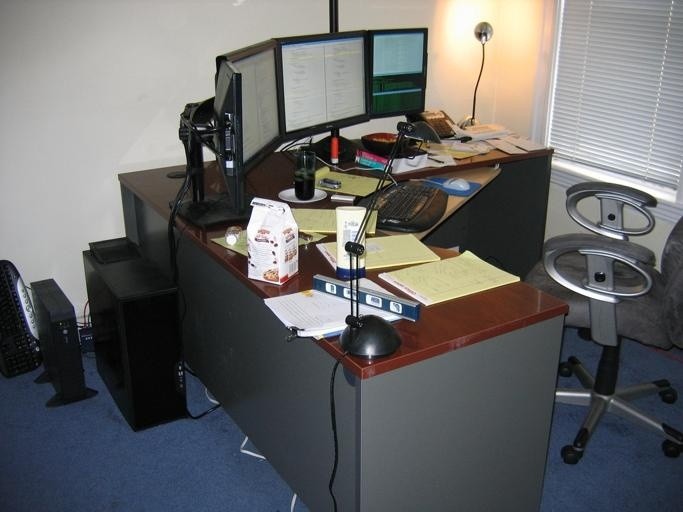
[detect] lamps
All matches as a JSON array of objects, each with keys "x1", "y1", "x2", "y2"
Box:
[
  {"x1": 337, "y1": 119, "x2": 442, "y2": 361},
  {"x1": 457, "y1": 20, "x2": 495, "y2": 130}
]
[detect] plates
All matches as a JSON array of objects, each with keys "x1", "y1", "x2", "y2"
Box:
[
  {"x1": 224, "y1": 225, "x2": 243, "y2": 247},
  {"x1": 278, "y1": 187, "x2": 328, "y2": 205}
]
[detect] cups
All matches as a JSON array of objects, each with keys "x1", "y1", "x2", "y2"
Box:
[{"x1": 294, "y1": 149, "x2": 316, "y2": 201}]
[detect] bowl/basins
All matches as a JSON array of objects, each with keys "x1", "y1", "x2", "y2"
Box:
[{"x1": 361, "y1": 132, "x2": 400, "y2": 155}]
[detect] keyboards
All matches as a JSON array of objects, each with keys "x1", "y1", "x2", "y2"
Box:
[
  {"x1": 0, "y1": 259, "x2": 42, "y2": 380},
  {"x1": 355, "y1": 181, "x2": 450, "y2": 235}
]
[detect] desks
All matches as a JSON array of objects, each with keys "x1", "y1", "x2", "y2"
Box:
[{"x1": 117, "y1": 123, "x2": 571, "y2": 512}]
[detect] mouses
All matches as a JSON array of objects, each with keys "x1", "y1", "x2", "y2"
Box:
[{"x1": 441, "y1": 177, "x2": 470, "y2": 192}]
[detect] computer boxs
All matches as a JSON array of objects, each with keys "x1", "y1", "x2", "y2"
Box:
[{"x1": 81, "y1": 242, "x2": 193, "y2": 434}]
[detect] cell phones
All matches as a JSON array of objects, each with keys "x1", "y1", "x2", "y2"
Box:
[{"x1": 320, "y1": 177, "x2": 342, "y2": 189}]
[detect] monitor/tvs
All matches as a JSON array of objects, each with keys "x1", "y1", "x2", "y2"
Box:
[
  {"x1": 169, "y1": 58, "x2": 260, "y2": 231},
  {"x1": 213, "y1": 38, "x2": 286, "y2": 180},
  {"x1": 278, "y1": 30, "x2": 371, "y2": 143},
  {"x1": 367, "y1": 27, "x2": 429, "y2": 120}
]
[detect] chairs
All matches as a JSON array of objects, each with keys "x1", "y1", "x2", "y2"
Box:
[{"x1": 523, "y1": 181, "x2": 683, "y2": 466}]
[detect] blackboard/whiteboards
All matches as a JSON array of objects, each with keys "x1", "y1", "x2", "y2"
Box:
[{"x1": 406, "y1": 111, "x2": 456, "y2": 139}]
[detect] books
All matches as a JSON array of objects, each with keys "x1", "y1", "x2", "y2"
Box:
[
  {"x1": 464, "y1": 123, "x2": 509, "y2": 141},
  {"x1": 348, "y1": 139, "x2": 428, "y2": 174}
]
